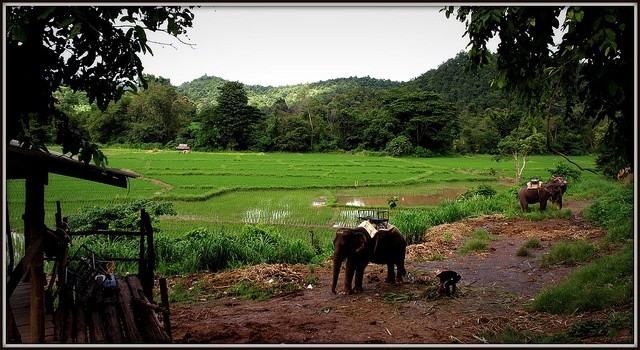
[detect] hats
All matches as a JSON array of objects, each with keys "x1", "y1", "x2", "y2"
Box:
[{"x1": 433, "y1": 269, "x2": 442, "y2": 278}]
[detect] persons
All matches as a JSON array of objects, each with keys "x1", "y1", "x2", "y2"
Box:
[{"x1": 433, "y1": 269, "x2": 461, "y2": 296}]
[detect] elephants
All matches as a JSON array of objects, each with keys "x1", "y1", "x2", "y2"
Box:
[
  {"x1": 330, "y1": 225, "x2": 408, "y2": 295},
  {"x1": 547, "y1": 181, "x2": 567, "y2": 205},
  {"x1": 513, "y1": 184, "x2": 562, "y2": 213}
]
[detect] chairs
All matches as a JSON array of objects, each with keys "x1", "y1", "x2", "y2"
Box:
[
  {"x1": 530, "y1": 178, "x2": 540, "y2": 187},
  {"x1": 358, "y1": 209, "x2": 389, "y2": 230}
]
